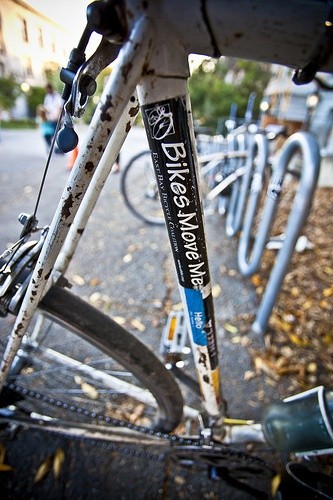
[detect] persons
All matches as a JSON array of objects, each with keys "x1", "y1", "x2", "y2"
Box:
[
  {"x1": 42, "y1": 83, "x2": 66, "y2": 123},
  {"x1": 37, "y1": 105, "x2": 60, "y2": 156}
]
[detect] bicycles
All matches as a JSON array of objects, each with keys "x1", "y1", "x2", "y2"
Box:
[{"x1": 0, "y1": 1, "x2": 333, "y2": 500}]
